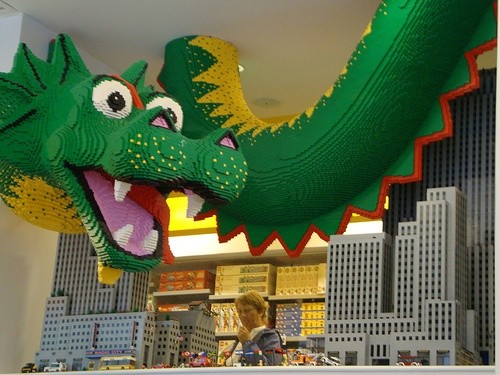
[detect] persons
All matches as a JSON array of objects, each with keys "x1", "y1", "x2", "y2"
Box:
[{"x1": 226, "y1": 290, "x2": 283, "y2": 366}]
